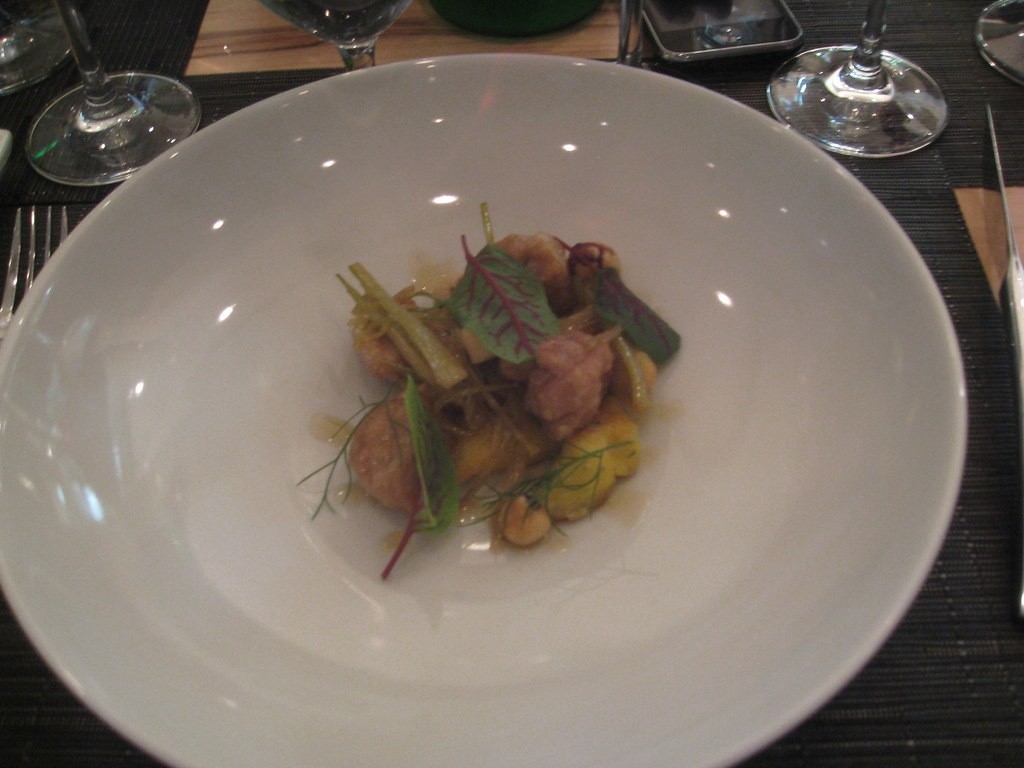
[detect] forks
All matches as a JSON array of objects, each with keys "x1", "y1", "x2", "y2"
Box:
[{"x1": 1, "y1": 205, "x2": 69, "y2": 333}]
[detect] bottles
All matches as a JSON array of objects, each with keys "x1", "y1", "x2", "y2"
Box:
[{"x1": 429, "y1": 0, "x2": 603, "y2": 36}]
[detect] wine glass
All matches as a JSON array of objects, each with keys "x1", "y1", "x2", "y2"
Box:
[
  {"x1": 766, "y1": 0, "x2": 947, "y2": 158},
  {"x1": 973, "y1": 0, "x2": 1024, "y2": 87},
  {"x1": 257, "y1": 0, "x2": 415, "y2": 70},
  {"x1": 0, "y1": 0, "x2": 202, "y2": 186}
]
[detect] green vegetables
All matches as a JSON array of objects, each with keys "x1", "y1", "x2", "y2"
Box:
[{"x1": 299, "y1": 204, "x2": 683, "y2": 580}]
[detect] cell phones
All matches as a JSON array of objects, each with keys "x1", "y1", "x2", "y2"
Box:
[{"x1": 640, "y1": 0, "x2": 805, "y2": 78}]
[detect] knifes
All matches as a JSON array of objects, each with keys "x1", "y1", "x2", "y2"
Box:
[{"x1": 985, "y1": 103, "x2": 1024, "y2": 476}]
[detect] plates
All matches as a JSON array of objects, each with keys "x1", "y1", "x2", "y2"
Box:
[{"x1": 0, "y1": 52, "x2": 966, "y2": 768}]
